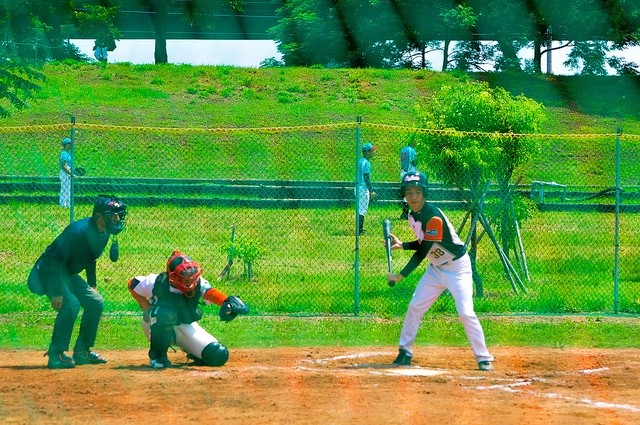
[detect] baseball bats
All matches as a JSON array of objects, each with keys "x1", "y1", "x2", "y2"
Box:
[{"x1": 383, "y1": 219, "x2": 395, "y2": 287}]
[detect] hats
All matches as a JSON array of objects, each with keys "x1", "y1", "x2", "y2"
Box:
[
  {"x1": 62, "y1": 138, "x2": 76, "y2": 146},
  {"x1": 362, "y1": 142, "x2": 377, "y2": 152}
]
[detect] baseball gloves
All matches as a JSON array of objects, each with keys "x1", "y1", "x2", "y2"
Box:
[
  {"x1": 74, "y1": 167, "x2": 85, "y2": 176},
  {"x1": 220, "y1": 295, "x2": 249, "y2": 322}
]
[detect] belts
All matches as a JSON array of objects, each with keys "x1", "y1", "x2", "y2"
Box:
[{"x1": 441, "y1": 248, "x2": 466, "y2": 265}]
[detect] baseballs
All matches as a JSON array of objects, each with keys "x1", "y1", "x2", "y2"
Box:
[{"x1": 104, "y1": 276, "x2": 111, "y2": 283}]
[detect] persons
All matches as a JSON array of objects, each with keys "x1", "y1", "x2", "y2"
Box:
[
  {"x1": 58, "y1": 138, "x2": 71, "y2": 207},
  {"x1": 38, "y1": 194, "x2": 127, "y2": 368},
  {"x1": 94, "y1": 44, "x2": 108, "y2": 63},
  {"x1": 354, "y1": 142, "x2": 378, "y2": 234},
  {"x1": 400, "y1": 139, "x2": 419, "y2": 219},
  {"x1": 128, "y1": 250, "x2": 248, "y2": 368},
  {"x1": 380, "y1": 171, "x2": 495, "y2": 370}
]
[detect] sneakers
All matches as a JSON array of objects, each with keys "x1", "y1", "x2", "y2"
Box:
[
  {"x1": 47, "y1": 352, "x2": 75, "y2": 368},
  {"x1": 149, "y1": 357, "x2": 170, "y2": 368},
  {"x1": 73, "y1": 350, "x2": 107, "y2": 365},
  {"x1": 479, "y1": 361, "x2": 492, "y2": 370},
  {"x1": 392, "y1": 349, "x2": 411, "y2": 366}
]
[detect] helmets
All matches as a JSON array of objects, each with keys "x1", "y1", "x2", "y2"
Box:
[
  {"x1": 400, "y1": 172, "x2": 429, "y2": 198},
  {"x1": 92, "y1": 194, "x2": 127, "y2": 235},
  {"x1": 166, "y1": 250, "x2": 202, "y2": 293}
]
